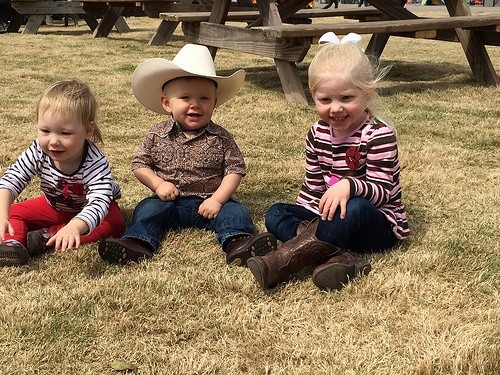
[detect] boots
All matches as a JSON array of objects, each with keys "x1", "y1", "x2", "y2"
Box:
[
  {"x1": 247, "y1": 216, "x2": 340, "y2": 289},
  {"x1": 295, "y1": 220, "x2": 371, "y2": 291}
]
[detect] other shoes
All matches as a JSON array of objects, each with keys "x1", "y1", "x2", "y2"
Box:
[
  {"x1": 98, "y1": 237, "x2": 153, "y2": 266},
  {"x1": 0, "y1": 241, "x2": 28, "y2": 268},
  {"x1": 226, "y1": 233, "x2": 277, "y2": 267},
  {"x1": 27, "y1": 228, "x2": 55, "y2": 258}
]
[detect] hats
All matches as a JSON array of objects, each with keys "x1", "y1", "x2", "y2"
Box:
[{"x1": 132, "y1": 43, "x2": 246, "y2": 115}]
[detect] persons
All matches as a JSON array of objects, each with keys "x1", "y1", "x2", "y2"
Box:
[
  {"x1": 323, "y1": 0, "x2": 371, "y2": 10},
  {"x1": 247, "y1": 31, "x2": 409, "y2": 291},
  {"x1": 0, "y1": 79, "x2": 124, "y2": 267},
  {"x1": 98, "y1": 44, "x2": 277, "y2": 267}
]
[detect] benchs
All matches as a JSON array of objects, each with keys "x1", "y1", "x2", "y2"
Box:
[
  {"x1": 250, "y1": 13, "x2": 500, "y2": 39},
  {"x1": 159, "y1": 6, "x2": 407, "y2": 22}
]
